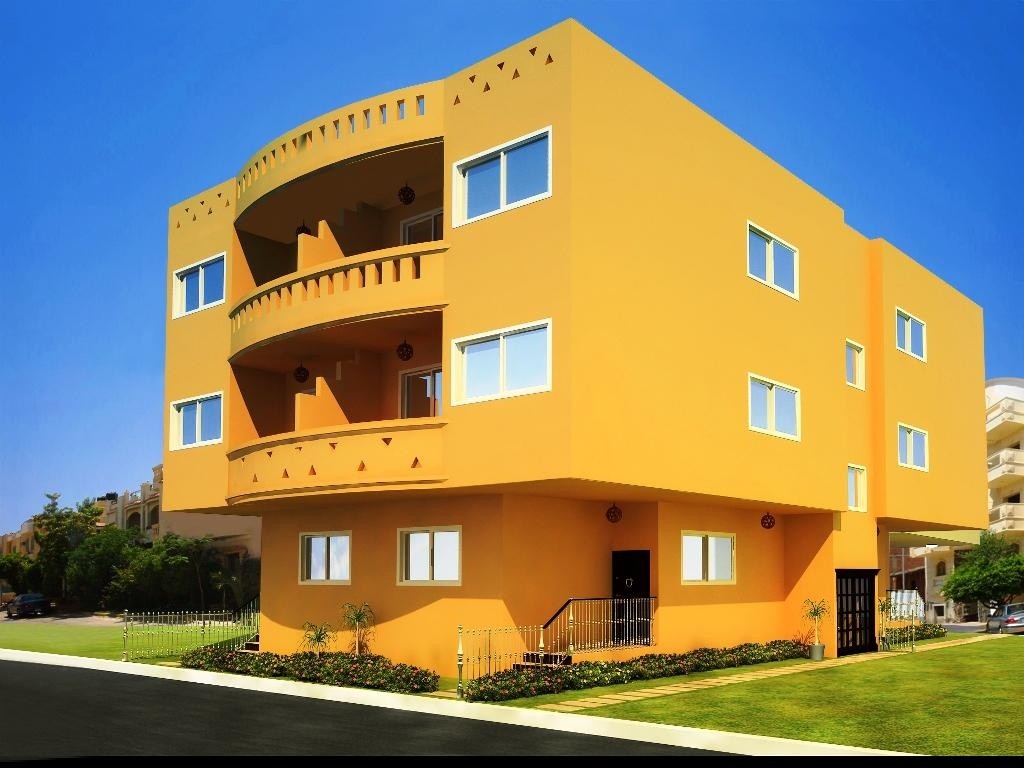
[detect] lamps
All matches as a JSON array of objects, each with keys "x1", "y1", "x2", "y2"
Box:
[
  {"x1": 293, "y1": 361, "x2": 310, "y2": 382},
  {"x1": 295, "y1": 221, "x2": 311, "y2": 237},
  {"x1": 399, "y1": 185, "x2": 415, "y2": 204},
  {"x1": 605, "y1": 502, "x2": 624, "y2": 524},
  {"x1": 396, "y1": 336, "x2": 413, "y2": 361},
  {"x1": 761, "y1": 512, "x2": 777, "y2": 528}
]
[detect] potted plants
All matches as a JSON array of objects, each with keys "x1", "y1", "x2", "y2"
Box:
[{"x1": 801, "y1": 598, "x2": 831, "y2": 660}]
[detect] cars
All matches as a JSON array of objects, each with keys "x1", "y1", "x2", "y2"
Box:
[
  {"x1": 4, "y1": 592, "x2": 59, "y2": 621},
  {"x1": 985, "y1": 602, "x2": 1024, "y2": 635}
]
